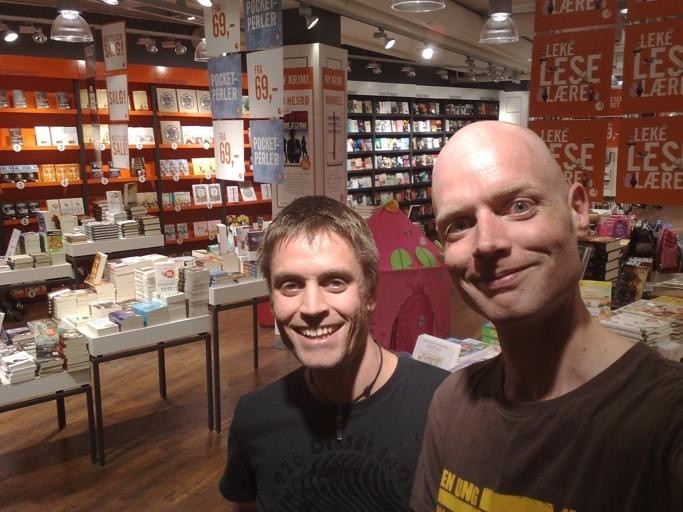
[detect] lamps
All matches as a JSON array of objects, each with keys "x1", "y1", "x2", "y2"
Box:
[
  {"x1": 299, "y1": 5, "x2": 319, "y2": 31},
  {"x1": 479, "y1": 0, "x2": 519, "y2": 44},
  {"x1": 1, "y1": 24, "x2": 18, "y2": 42},
  {"x1": 373, "y1": 26, "x2": 395, "y2": 51},
  {"x1": 198, "y1": 0, "x2": 212, "y2": 7},
  {"x1": 49, "y1": 0, "x2": 95, "y2": 43}
]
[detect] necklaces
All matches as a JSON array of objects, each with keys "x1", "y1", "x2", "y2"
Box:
[{"x1": 310, "y1": 340, "x2": 384, "y2": 442}]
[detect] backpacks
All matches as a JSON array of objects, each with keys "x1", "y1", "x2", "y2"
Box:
[
  {"x1": 657, "y1": 224, "x2": 682, "y2": 273},
  {"x1": 633, "y1": 219, "x2": 657, "y2": 257}
]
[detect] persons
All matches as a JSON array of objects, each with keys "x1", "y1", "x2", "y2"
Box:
[
  {"x1": 217, "y1": 195, "x2": 454, "y2": 512},
  {"x1": 409, "y1": 120, "x2": 683, "y2": 512}
]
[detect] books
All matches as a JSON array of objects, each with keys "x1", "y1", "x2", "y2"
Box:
[
  {"x1": 0, "y1": 85, "x2": 271, "y2": 383},
  {"x1": 411, "y1": 320, "x2": 502, "y2": 373},
  {"x1": 345, "y1": 96, "x2": 500, "y2": 239},
  {"x1": 578, "y1": 201, "x2": 682, "y2": 374}
]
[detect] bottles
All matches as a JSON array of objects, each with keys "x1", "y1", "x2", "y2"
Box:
[{"x1": 3, "y1": 202, "x2": 39, "y2": 219}]
